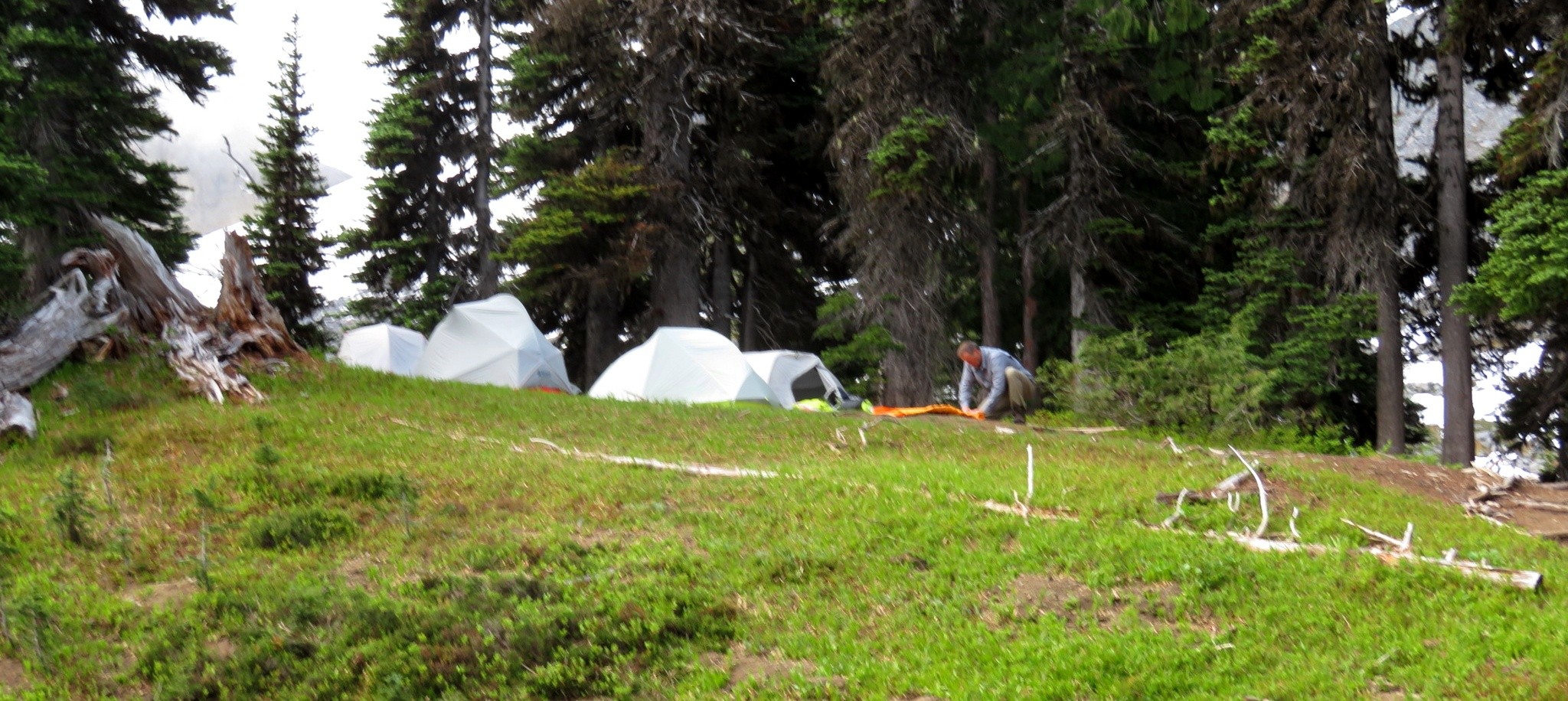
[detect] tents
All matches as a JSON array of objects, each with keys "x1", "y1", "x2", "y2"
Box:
[
  {"x1": 587, "y1": 326, "x2": 783, "y2": 408},
  {"x1": 417, "y1": 292, "x2": 579, "y2": 395},
  {"x1": 742, "y1": 350, "x2": 850, "y2": 410},
  {"x1": 336, "y1": 322, "x2": 428, "y2": 377}
]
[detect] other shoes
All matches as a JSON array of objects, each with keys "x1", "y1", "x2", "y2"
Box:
[{"x1": 1013, "y1": 412, "x2": 1027, "y2": 424}]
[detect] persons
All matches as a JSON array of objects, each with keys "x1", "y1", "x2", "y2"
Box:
[{"x1": 956, "y1": 342, "x2": 1037, "y2": 424}]
